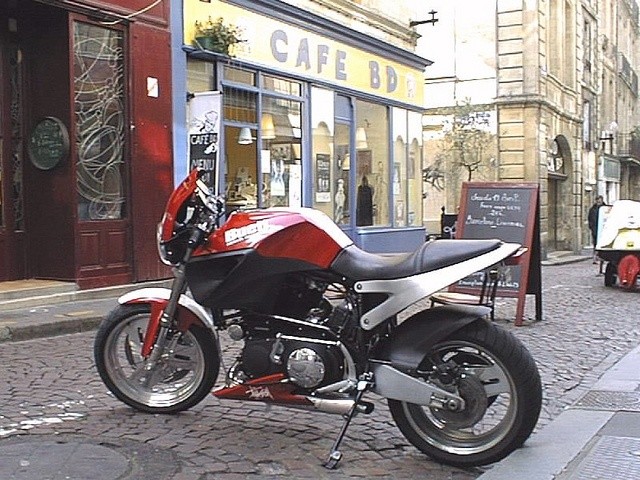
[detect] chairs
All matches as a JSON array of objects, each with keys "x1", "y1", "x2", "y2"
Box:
[
  {"x1": 429, "y1": 260, "x2": 502, "y2": 321},
  {"x1": 426, "y1": 206, "x2": 460, "y2": 240}
]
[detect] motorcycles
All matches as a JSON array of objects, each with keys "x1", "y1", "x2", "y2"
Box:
[{"x1": 93, "y1": 168, "x2": 543, "y2": 469}]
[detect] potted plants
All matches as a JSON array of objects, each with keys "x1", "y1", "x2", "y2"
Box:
[{"x1": 195, "y1": 15, "x2": 247, "y2": 58}]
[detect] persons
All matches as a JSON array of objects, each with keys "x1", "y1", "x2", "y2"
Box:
[{"x1": 587, "y1": 195, "x2": 606, "y2": 264}]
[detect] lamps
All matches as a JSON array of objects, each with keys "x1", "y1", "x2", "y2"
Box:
[
  {"x1": 238, "y1": 127, "x2": 258, "y2": 145},
  {"x1": 355, "y1": 116, "x2": 372, "y2": 149},
  {"x1": 262, "y1": 113, "x2": 277, "y2": 140},
  {"x1": 340, "y1": 153, "x2": 350, "y2": 171}
]
